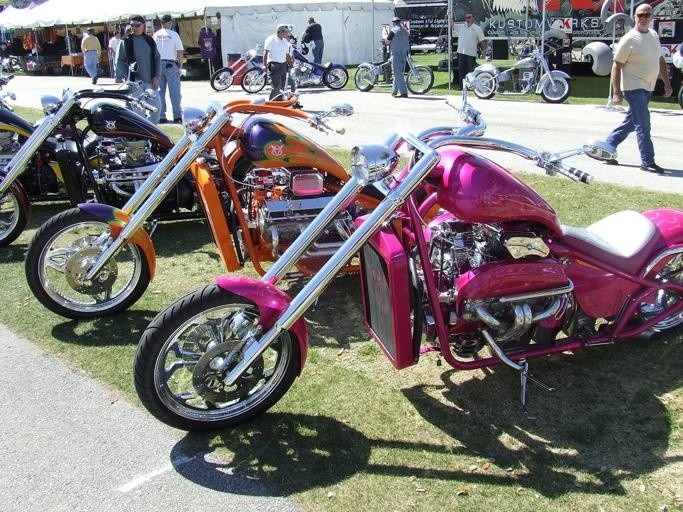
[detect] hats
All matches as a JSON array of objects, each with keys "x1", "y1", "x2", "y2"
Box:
[
  {"x1": 160, "y1": 14, "x2": 173, "y2": 23},
  {"x1": 276, "y1": 25, "x2": 293, "y2": 33},
  {"x1": 128, "y1": 16, "x2": 145, "y2": 23},
  {"x1": 126, "y1": 24, "x2": 132, "y2": 30},
  {"x1": 392, "y1": 17, "x2": 402, "y2": 22}
]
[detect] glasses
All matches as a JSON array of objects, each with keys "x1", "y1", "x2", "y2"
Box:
[
  {"x1": 127, "y1": 28, "x2": 131, "y2": 31},
  {"x1": 636, "y1": 13, "x2": 656, "y2": 19},
  {"x1": 465, "y1": 16, "x2": 472, "y2": 19},
  {"x1": 130, "y1": 24, "x2": 142, "y2": 28}
]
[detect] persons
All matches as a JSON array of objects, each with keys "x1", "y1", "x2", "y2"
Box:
[
  {"x1": 384, "y1": 17, "x2": 409, "y2": 97},
  {"x1": 604, "y1": 4, "x2": 673, "y2": 174},
  {"x1": 113, "y1": 14, "x2": 162, "y2": 125},
  {"x1": 64, "y1": 30, "x2": 75, "y2": 54},
  {"x1": 81, "y1": 27, "x2": 101, "y2": 84},
  {"x1": 284, "y1": 71, "x2": 302, "y2": 109},
  {"x1": 263, "y1": 25, "x2": 293, "y2": 102},
  {"x1": 152, "y1": 14, "x2": 185, "y2": 122},
  {"x1": 300, "y1": 17, "x2": 323, "y2": 72},
  {"x1": 448, "y1": 10, "x2": 487, "y2": 90},
  {"x1": 109, "y1": 30, "x2": 123, "y2": 78}
]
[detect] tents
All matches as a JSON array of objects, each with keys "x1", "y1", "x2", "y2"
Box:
[{"x1": 1, "y1": 3, "x2": 395, "y2": 82}]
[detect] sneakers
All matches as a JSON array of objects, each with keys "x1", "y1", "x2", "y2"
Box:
[
  {"x1": 115, "y1": 80, "x2": 123, "y2": 83},
  {"x1": 93, "y1": 76, "x2": 97, "y2": 83},
  {"x1": 173, "y1": 118, "x2": 183, "y2": 122},
  {"x1": 158, "y1": 119, "x2": 168, "y2": 124},
  {"x1": 291, "y1": 103, "x2": 303, "y2": 109}
]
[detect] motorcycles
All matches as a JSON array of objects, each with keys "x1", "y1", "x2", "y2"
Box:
[
  {"x1": 355, "y1": 49, "x2": 434, "y2": 95},
  {"x1": 0, "y1": 84, "x2": 213, "y2": 248},
  {"x1": 210, "y1": 49, "x2": 276, "y2": 89},
  {"x1": 240, "y1": 33, "x2": 348, "y2": 92},
  {"x1": 472, "y1": 37, "x2": 572, "y2": 103},
  {"x1": 24, "y1": 90, "x2": 441, "y2": 318},
  {"x1": 133, "y1": 73, "x2": 683, "y2": 432}
]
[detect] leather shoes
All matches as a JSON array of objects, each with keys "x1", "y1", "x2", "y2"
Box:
[
  {"x1": 395, "y1": 94, "x2": 408, "y2": 98},
  {"x1": 604, "y1": 159, "x2": 619, "y2": 166},
  {"x1": 642, "y1": 164, "x2": 667, "y2": 174}
]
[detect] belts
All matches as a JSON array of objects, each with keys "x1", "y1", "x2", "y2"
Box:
[
  {"x1": 159, "y1": 58, "x2": 179, "y2": 64},
  {"x1": 85, "y1": 49, "x2": 98, "y2": 52},
  {"x1": 270, "y1": 61, "x2": 281, "y2": 65}
]
